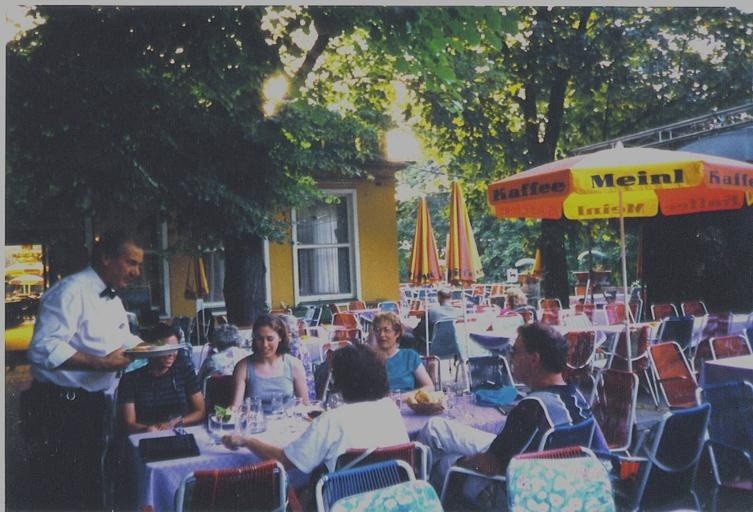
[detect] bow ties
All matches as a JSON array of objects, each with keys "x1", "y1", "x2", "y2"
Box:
[{"x1": 99, "y1": 287, "x2": 118, "y2": 299}]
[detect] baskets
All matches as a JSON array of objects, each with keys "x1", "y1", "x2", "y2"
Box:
[{"x1": 410, "y1": 403, "x2": 444, "y2": 414}]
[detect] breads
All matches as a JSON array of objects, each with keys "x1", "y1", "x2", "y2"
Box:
[{"x1": 406, "y1": 389, "x2": 444, "y2": 404}]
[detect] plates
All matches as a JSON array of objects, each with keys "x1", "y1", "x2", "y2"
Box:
[{"x1": 126, "y1": 344, "x2": 186, "y2": 357}]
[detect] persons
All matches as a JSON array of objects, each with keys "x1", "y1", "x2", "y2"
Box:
[
  {"x1": 26, "y1": 231, "x2": 162, "y2": 512},
  {"x1": 230, "y1": 316, "x2": 310, "y2": 411},
  {"x1": 196, "y1": 323, "x2": 253, "y2": 393},
  {"x1": 118, "y1": 326, "x2": 206, "y2": 433},
  {"x1": 220, "y1": 344, "x2": 411, "y2": 475},
  {"x1": 371, "y1": 312, "x2": 435, "y2": 403},
  {"x1": 413, "y1": 322, "x2": 591, "y2": 512},
  {"x1": 400, "y1": 288, "x2": 457, "y2": 344}
]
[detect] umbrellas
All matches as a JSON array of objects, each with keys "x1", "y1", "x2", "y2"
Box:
[
  {"x1": 408, "y1": 195, "x2": 443, "y2": 357},
  {"x1": 9, "y1": 275, "x2": 46, "y2": 295},
  {"x1": 184, "y1": 256, "x2": 210, "y2": 345},
  {"x1": 6, "y1": 262, "x2": 45, "y2": 293},
  {"x1": 488, "y1": 140, "x2": 753, "y2": 372},
  {"x1": 448, "y1": 176, "x2": 485, "y2": 356}
]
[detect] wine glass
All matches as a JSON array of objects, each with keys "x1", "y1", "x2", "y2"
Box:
[{"x1": 212, "y1": 388, "x2": 475, "y2": 440}]
